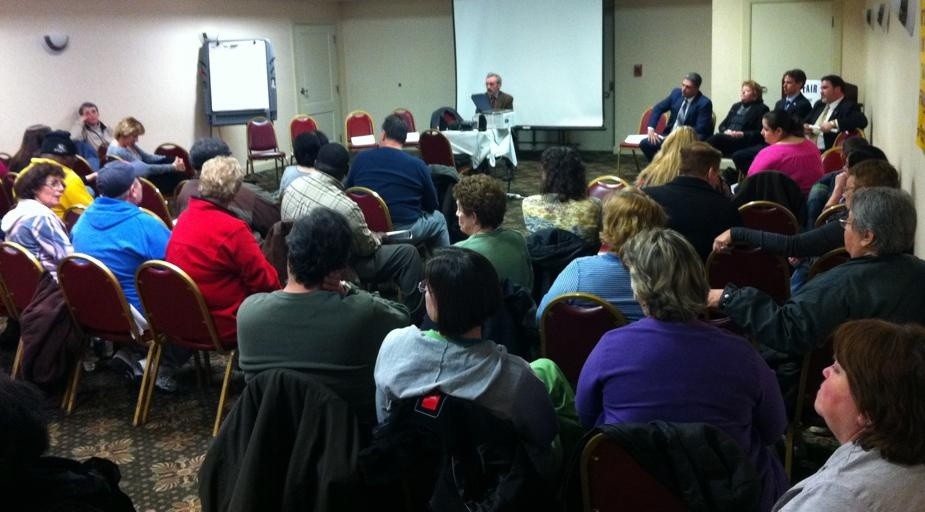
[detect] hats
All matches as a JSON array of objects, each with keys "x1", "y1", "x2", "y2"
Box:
[{"x1": 40, "y1": 130, "x2": 76, "y2": 155}]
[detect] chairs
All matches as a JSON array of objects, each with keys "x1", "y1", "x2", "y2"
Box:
[
  {"x1": 57, "y1": 253, "x2": 211, "y2": 423},
  {"x1": 135, "y1": 259, "x2": 239, "y2": 438},
  {"x1": 0, "y1": 240, "x2": 137, "y2": 415},
  {"x1": 382, "y1": 394, "x2": 574, "y2": 510},
  {"x1": 198, "y1": 368, "x2": 382, "y2": 510}
]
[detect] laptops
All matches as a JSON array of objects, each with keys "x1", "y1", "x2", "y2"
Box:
[{"x1": 471, "y1": 92, "x2": 513, "y2": 114}]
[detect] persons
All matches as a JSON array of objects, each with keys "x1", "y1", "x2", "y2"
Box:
[
  {"x1": 471, "y1": 72, "x2": 514, "y2": 131},
  {"x1": 803, "y1": 74, "x2": 871, "y2": 149},
  {"x1": 710, "y1": 80, "x2": 769, "y2": 158},
  {"x1": 638, "y1": 71, "x2": 713, "y2": 162},
  {"x1": 774, "y1": 69, "x2": 812, "y2": 120},
  {"x1": 0, "y1": 100, "x2": 924, "y2": 512}
]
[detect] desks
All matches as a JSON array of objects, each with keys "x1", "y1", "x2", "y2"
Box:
[{"x1": 439, "y1": 128, "x2": 518, "y2": 185}]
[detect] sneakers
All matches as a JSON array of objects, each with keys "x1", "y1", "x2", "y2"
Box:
[
  {"x1": 111, "y1": 350, "x2": 144, "y2": 400},
  {"x1": 137, "y1": 357, "x2": 178, "y2": 392}
]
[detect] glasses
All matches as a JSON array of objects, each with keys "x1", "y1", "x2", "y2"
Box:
[
  {"x1": 40, "y1": 181, "x2": 66, "y2": 191},
  {"x1": 419, "y1": 280, "x2": 433, "y2": 297},
  {"x1": 840, "y1": 185, "x2": 856, "y2": 193},
  {"x1": 838, "y1": 216, "x2": 854, "y2": 227}
]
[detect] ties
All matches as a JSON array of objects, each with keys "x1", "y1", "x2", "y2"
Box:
[
  {"x1": 491, "y1": 95, "x2": 496, "y2": 109},
  {"x1": 813, "y1": 105, "x2": 830, "y2": 144},
  {"x1": 785, "y1": 100, "x2": 789, "y2": 110},
  {"x1": 676, "y1": 100, "x2": 688, "y2": 126}
]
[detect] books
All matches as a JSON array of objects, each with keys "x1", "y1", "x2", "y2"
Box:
[{"x1": 472, "y1": 93, "x2": 492, "y2": 114}]
